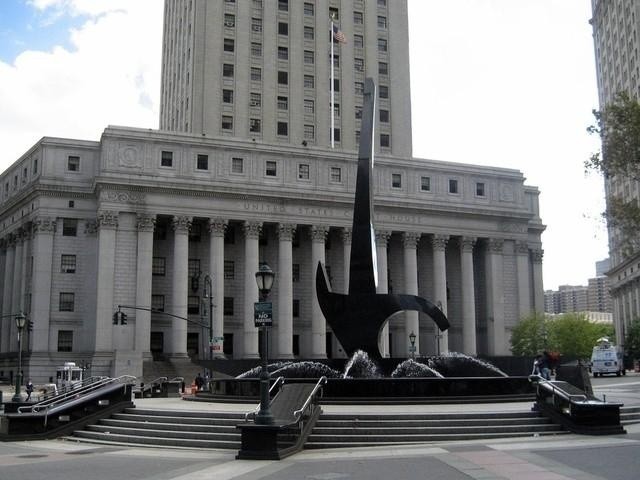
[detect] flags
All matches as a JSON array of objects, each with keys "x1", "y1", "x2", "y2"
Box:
[{"x1": 330, "y1": 17, "x2": 347, "y2": 44}]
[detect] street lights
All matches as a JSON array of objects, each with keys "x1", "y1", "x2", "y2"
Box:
[
  {"x1": 255, "y1": 264, "x2": 275, "y2": 425},
  {"x1": 11, "y1": 309, "x2": 26, "y2": 401},
  {"x1": 409, "y1": 330, "x2": 416, "y2": 361},
  {"x1": 203, "y1": 274, "x2": 213, "y2": 378},
  {"x1": 541, "y1": 330, "x2": 548, "y2": 351}
]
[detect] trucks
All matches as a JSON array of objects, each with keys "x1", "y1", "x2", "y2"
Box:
[{"x1": 591, "y1": 337, "x2": 626, "y2": 377}]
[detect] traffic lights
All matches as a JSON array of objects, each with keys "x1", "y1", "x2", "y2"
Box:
[
  {"x1": 28, "y1": 318, "x2": 33, "y2": 332},
  {"x1": 112, "y1": 311, "x2": 128, "y2": 325}
]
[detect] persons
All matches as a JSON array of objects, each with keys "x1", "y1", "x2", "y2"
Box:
[
  {"x1": 24, "y1": 378, "x2": 33, "y2": 402},
  {"x1": 531, "y1": 351, "x2": 553, "y2": 381},
  {"x1": 195, "y1": 373, "x2": 204, "y2": 390}
]
